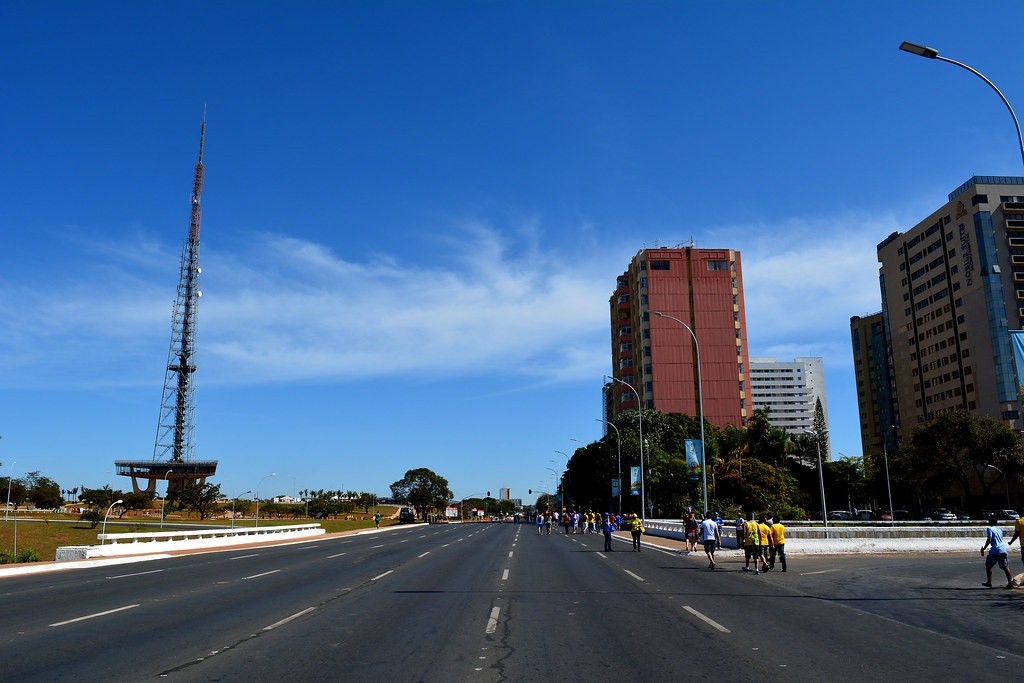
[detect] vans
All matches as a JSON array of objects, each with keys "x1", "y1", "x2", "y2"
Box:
[{"x1": 399, "y1": 507, "x2": 415, "y2": 524}]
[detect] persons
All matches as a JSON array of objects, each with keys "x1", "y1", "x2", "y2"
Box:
[
  {"x1": 536, "y1": 512, "x2": 551, "y2": 536},
  {"x1": 602, "y1": 513, "x2": 614, "y2": 552},
  {"x1": 742, "y1": 512, "x2": 787, "y2": 575},
  {"x1": 428, "y1": 512, "x2": 448, "y2": 524},
  {"x1": 629, "y1": 513, "x2": 642, "y2": 552},
  {"x1": 735, "y1": 513, "x2": 745, "y2": 549},
  {"x1": 1008, "y1": 507, "x2": 1024, "y2": 568},
  {"x1": 699, "y1": 512, "x2": 720, "y2": 570},
  {"x1": 564, "y1": 510, "x2": 621, "y2": 535},
  {"x1": 526, "y1": 509, "x2": 559, "y2": 527},
  {"x1": 375, "y1": 511, "x2": 380, "y2": 529},
  {"x1": 514, "y1": 511, "x2": 520, "y2": 524},
  {"x1": 980, "y1": 516, "x2": 1013, "y2": 589},
  {"x1": 683, "y1": 514, "x2": 699, "y2": 552},
  {"x1": 699, "y1": 513, "x2": 724, "y2": 550},
  {"x1": 499, "y1": 511, "x2": 503, "y2": 523}
]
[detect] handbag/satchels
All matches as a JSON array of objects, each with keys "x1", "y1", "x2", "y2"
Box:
[
  {"x1": 639, "y1": 525, "x2": 646, "y2": 533},
  {"x1": 610, "y1": 523, "x2": 615, "y2": 532}
]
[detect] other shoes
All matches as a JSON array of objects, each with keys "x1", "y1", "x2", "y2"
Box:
[
  {"x1": 605, "y1": 549, "x2": 614, "y2": 552},
  {"x1": 763, "y1": 565, "x2": 771, "y2": 573},
  {"x1": 768, "y1": 567, "x2": 774, "y2": 572},
  {"x1": 1004, "y1": 584, "x2": 1014, "y2": 589},
  {"x1": 742, "y1": 566, "x2": 751, "y2": 572},
  {"x1": 707, "y1": 562, "x2": 717, "y2": 570},
  {"x1": 781, "y1": 569, "x2": 788, "y2": 572},
  {"x1": 753, "y1": 571, "x2": 761, "y2": 576},
  {"x1": 982, "y1": 582, "x2": 992, "y2": 587},
  {"x1": 633, "y1": 549, "x2": 641, "y2": 552}
]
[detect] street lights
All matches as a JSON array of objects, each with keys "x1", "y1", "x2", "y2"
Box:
[
  {"x1": 102, "y1": 499, "x2": 124, "y2": 545},
  {"x1": 594, "y1": 418, "x2": 622, "y2": 532},
  {"x1": 897, "y1": 39, "x2": 1023, "y2": 167},
  {"x1": 231, "y1": 490, "x2": 253, "y2": 537},
  {"x1": 256, "y1": 473, "x2": 277, "y2": 527},
  {"x1": 5, "y1": 461, "x2": 17, "y2": 522},
  {"x1": 883, "y1": 424, "x2": 895, "y2": 527},
  {"x1": 654, "y1": 310, "x2": 708, "y2": 520},
  {"x1": 161, "y1": 469, "x2": 173, "y2": 528},
  {"x1": 803, "y1": 429, "x2": 829, "y2": 539},
  {"x1": 607, "y1": 374, "x2": 646, "y2": 529}
]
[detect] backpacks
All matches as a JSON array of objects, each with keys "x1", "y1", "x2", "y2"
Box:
[
  {"x1": 538, "y1": 515, "x2": 543, "y2": 523},
  {"x1": 588, "y1": 513, "x2": 593, "y2": 521},
  {"x1": 582, "y1": 515, "x2": 587, "y2": 522}
]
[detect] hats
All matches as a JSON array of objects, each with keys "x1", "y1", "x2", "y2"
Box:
[
  {"x1": 633, "y1": 514, "x2": 638, "y2": 518},
  {"x1": 603, "y1": 512, "x2": 610, "y2": 516},
  {"x1": 737, "y1": 512, "x2": 741, "y2": 515},
  {"x1": 715, "y1": 512, "x2": 718, "y2": 515}
]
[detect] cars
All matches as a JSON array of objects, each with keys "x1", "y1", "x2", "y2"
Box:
[{"x1": 827, "y1": 508, "x2": 1020, "y2": 521}]
